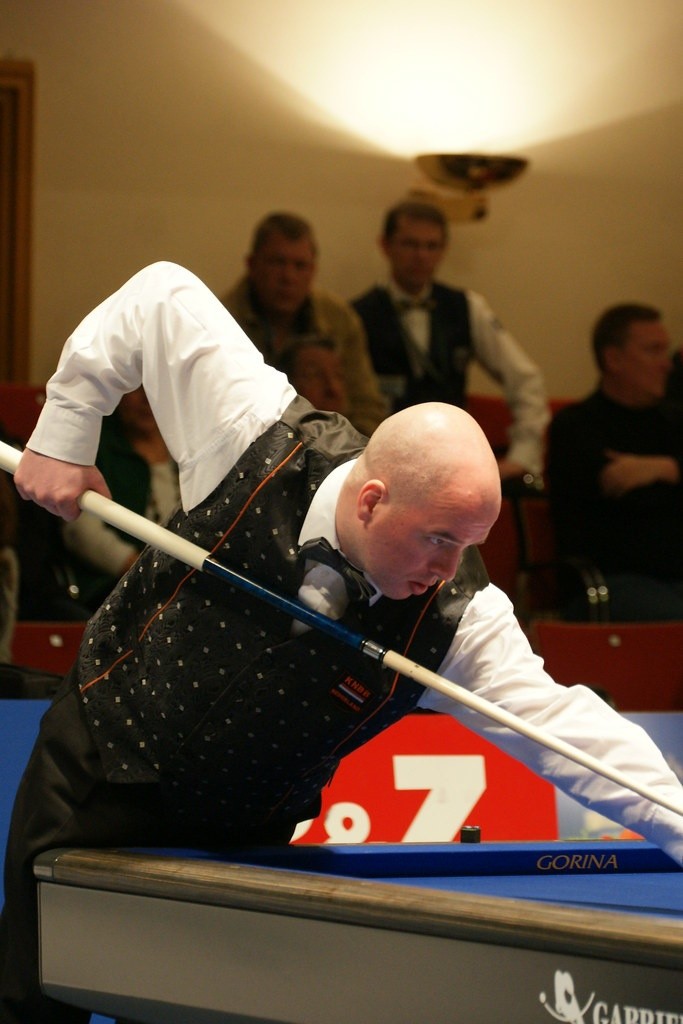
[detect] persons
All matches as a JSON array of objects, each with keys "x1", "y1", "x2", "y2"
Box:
[
  {"x1": 58, "y1": 383, "x2": 185, "y2": 613},
  {"x1": 221, "y1": 213, "x2": 386, "y2": 439},
  {"x1": 0, "y1": 263, "x2": 683, "y2": 1024},
  {"x1": 0, "y1": 424, "x2": 93, "y2": 620},
  {"x1": 351, "y1": 203, "x2": 552, "y2": 485},
  {"x1": 543, "y1": 302, "x2": 683, "y2": 621}
]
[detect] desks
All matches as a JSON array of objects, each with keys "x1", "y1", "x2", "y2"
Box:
[{"x1": 29, "y1": 839, "x2": 683, "y2": 1024}]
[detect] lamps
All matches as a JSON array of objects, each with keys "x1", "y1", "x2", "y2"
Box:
[{"x1": 418, "y1": 154, "x2": 530, "y2": 191}]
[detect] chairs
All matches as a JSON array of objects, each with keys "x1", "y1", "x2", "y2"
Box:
[{"x1": 477, "y1": 477, "x2": 612, "y2": 625}]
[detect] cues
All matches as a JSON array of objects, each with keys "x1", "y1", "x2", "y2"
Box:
[{"x1": 0, "y1": 441, "x2": 683, "y2": 821}]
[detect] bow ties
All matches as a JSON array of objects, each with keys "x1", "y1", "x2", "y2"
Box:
[
  {"x1": 394, "y1": 298, "x2": 437, "y2": 316},
  {"x1": 299, "y1": 536, "x2": 377, "y2": 603}
]
